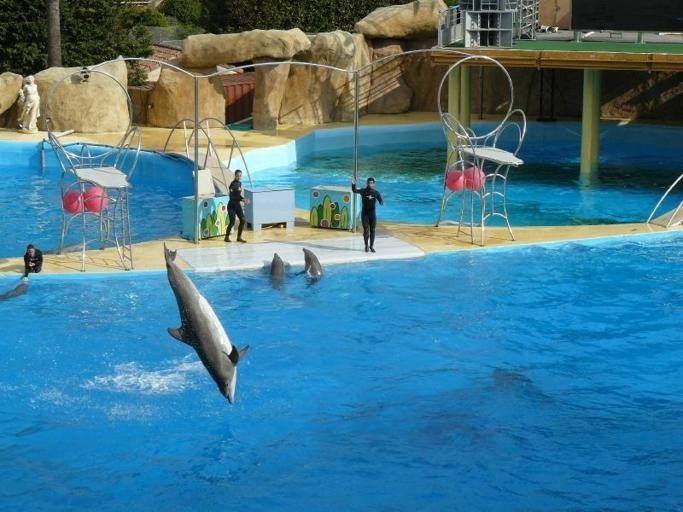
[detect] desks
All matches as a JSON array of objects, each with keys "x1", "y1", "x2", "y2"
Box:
[{"x1": 244, "y1": 185, "x2": 295, "y2": 234}]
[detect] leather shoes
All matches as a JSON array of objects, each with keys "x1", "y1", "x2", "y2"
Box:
[
  {"x1": 224, "y1": 237, "x2": 247, "y2": 243},
  {"x1": 365, "y1": 246, "x2": 376, "y2": 253}
]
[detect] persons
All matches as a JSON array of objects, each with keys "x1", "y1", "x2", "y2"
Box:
[
  {"x1": 224, "y1": 170, "x2": 248, "y2": 242},
  {"x1": 17, "y1": 75, "x2": 41, "y2": 134},
  {"x1": 352, "y1": 177, "x2": 383, "y2": 252},
  {"x1": 22, "y1": 244, "x2": 42, "y2": 281}
]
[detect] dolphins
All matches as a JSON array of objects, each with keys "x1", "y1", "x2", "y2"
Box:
[
  {"x1": 270, "y1": 252, "x2": 287, "y2": 292},
  {"x1": 0, "y1": 283, "x2": 30, "y2": 301},
  {"x1": 296, "y1": 245, "x2": 324, "y2": 288},
  {"x1": 158, "y1": 242, "x2": 249, "y2": 408}
]
